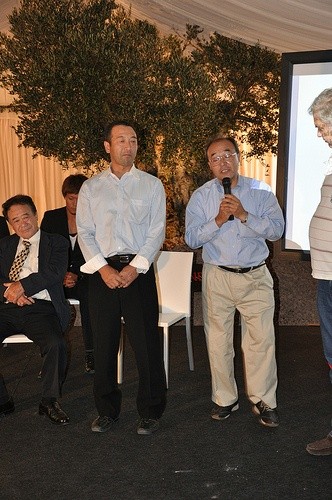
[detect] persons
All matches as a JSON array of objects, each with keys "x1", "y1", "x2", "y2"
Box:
[
  {"x1": 306, "y1": 88, "x2": 332, "y2": 456},
  {"x1": 0, "y1": 195, "x2": 70, "y2": 424},
  {"x1": 185, "y1": 138, "x2": 285, "y2": 426},
  {"x1": 40, "y1": 173, "x2": 95, "y2": 375},
  {"x1": 75, "y1": 121, "x2": 166, "y2": 434}
]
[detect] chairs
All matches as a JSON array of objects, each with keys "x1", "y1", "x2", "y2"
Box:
[
  {"x1": 2, "y1": 335, "x2": 45, "y2": 379},
  {"x1": 121, "y1": 251, "x2": 195, "y2": 389}
]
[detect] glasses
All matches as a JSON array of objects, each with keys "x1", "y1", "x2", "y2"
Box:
[{"x1": 209, "y1": 152, "x2": 237, "y2": 164}]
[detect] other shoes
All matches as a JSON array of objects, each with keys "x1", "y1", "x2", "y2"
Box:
[{"x1": 306, "y1": 431, "x2": 332, "y2": 455}]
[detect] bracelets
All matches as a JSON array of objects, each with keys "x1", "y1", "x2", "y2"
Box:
[{"x1": 241, "y1": 212, "x2": 248, "y2": 223}]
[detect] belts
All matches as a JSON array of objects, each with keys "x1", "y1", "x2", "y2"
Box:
[
  {"x1": 218, "y1": 263, "x2": 267, "y2": 274},
  {"x1": 107, "y1": 254, "x2": 138, "y2": 264}
]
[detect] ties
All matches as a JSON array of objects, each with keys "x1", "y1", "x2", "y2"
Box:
[{"x1": 8, "y1": 241, "x2": 32, "y2": 282}]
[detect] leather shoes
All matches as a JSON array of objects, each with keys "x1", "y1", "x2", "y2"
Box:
[
  {"x1": 1, "y1": 398, "x2": 16, "y2": 414},
  {"x1": 39, "y1": 400, "x2": 70, "y2": 424}
]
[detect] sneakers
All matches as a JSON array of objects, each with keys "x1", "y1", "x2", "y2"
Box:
[
  {"x1": 210, "y1": 402, "x2": 239, "y2": 420},
  {"x1": 252, "y1": 400, "x2": 280, "y2": 427},
  {"x1": 137, "y1": 418, "x2": 163, "y2": 436},
  {"x1": 91, "y1": 416, "x2": 120, "y2": 432}
]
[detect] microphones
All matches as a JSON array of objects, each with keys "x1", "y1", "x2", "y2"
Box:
[{"x1": 222, "y1": 177, "x2": 234, "y2": 220}]
